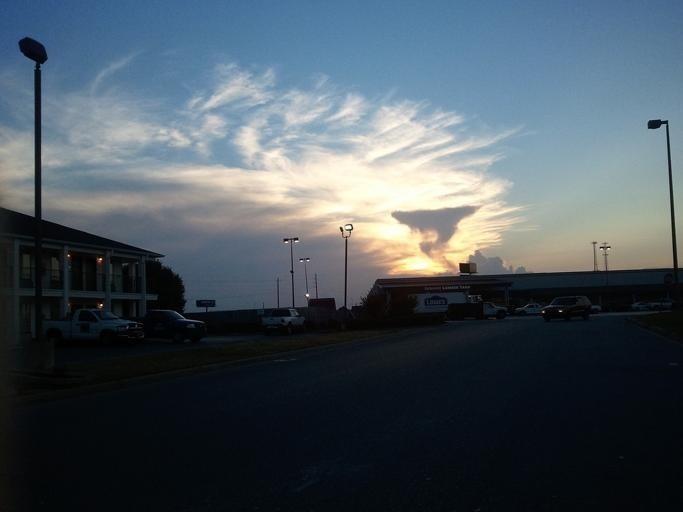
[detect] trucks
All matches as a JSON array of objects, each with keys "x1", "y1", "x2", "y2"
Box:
[
  {"x1": 303, "y1": 298, "x2": 336, "y2": 332},
  {"x1": 448, "y1": 300, "x2": 507, "y2": 321}
]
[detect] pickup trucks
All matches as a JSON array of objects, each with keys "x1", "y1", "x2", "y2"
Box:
[
  {"x1": 42, "y1": 309, "x2": 207, "y2": 348},
  {"x1": 261, "y1": 309, "x2": 305, "y2": 336}
]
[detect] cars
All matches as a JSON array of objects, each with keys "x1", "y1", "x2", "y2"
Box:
[
  {"x1": 632, "y1": 300, "x2": 671, "y2": 311},
  {"x1": 541, "y1": 296, "x2": 592, "y2": 320},
  {"x1": 513, "y1": 303, "x2": 542, "y2": 316}
]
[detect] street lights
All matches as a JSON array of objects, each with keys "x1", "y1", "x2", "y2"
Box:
[
  {"x1": 299, "y1": 258, "x2": 310, "y2": 301},
  {"x1": 648, "y1": 120, "x2": 678, "y2": 284},
  {"x1": 19, "y1": 37, "x2": 47, "y2": 337},
  {"x1": 600, "y1": 246, "x2": 611, "y2": 271},
  {"x1": 340, "y1": 224, "x2": 353, "y2": 307},
  {"x1": 284, "y1": 238, "x2": 299, "y2": 308},
  {"x1": 593, "y1": 242, "x2": 597, "y2": 271}
]
